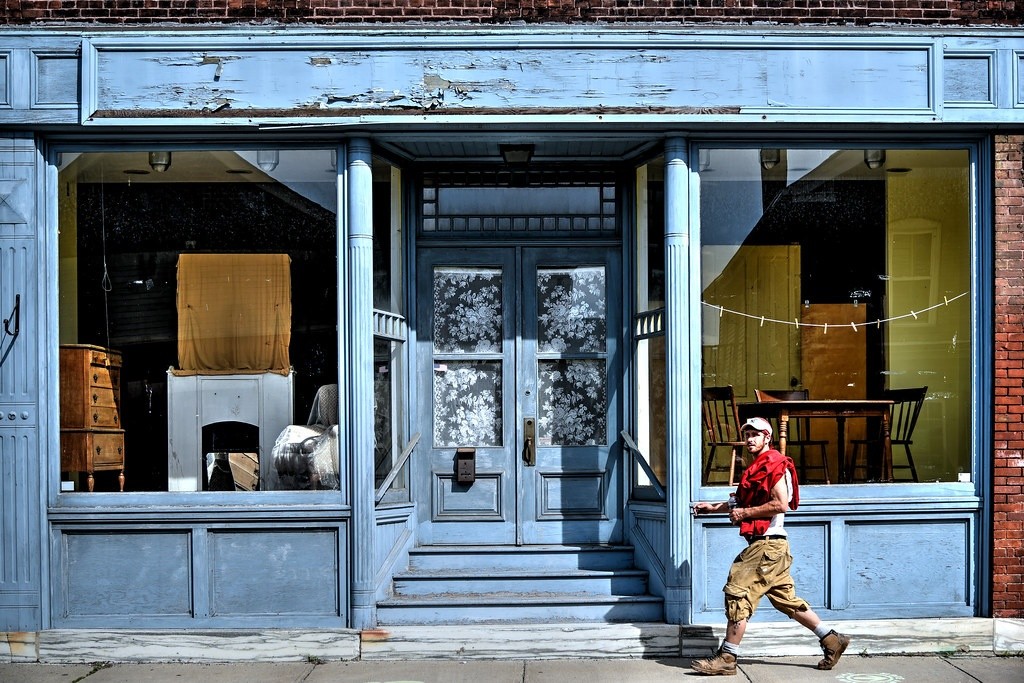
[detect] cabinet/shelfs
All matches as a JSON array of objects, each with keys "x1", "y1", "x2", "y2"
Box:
[{"x1": 59, "y1": 343, "x2": 125, "y2": 492}]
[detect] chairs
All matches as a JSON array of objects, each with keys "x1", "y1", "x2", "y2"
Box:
[
  {"x1": 754, "y1": 388, "x2": 831, "y2": 484},
  {"x1": 701, "y1": 385, "x2": 746, "y2": 487},
  {"x1": 849, "y1": 386, "x2": 929, "y2": 483}
]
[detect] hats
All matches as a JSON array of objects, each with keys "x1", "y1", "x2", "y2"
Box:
[{"x1": 740, "y1": 417, "x2": 772, "y2": 436}]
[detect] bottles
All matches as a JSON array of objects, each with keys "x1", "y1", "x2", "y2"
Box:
[{"x1": 727, "y1": 493, "x2": 740, "y2": 509}]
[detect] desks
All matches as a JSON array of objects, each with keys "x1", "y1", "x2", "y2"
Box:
[{"x1": 727, "y1": 400, "x2": 901, "y2": 484}]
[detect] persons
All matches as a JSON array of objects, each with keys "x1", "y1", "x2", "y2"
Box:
[{"x1": 688, "y1": 414, "x2": 850, "y2": 676}]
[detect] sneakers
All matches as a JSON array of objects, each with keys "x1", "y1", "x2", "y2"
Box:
[
  {"x1": 690, "y1": 646, "x2": 737, "y2": 675},
  {"x1": 818, "y1": 629, "x2": 850, "y2": 670}
]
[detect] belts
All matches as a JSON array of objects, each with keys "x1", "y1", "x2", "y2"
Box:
[{"x1": 748, "y1": 535, "x2": 786, "y2": 544}]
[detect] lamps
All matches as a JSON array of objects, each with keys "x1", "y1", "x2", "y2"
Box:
[
  {"x1": 148, "y1": 152, "x2": 171, "y2": 174},
  {"x1": 257, "y1": 151, "x2": 279, "y2": 172},
  {"x1": 758, "y1": 149, "x2": 780, "y2": 169},
  {"x1": 864, "y1": 150, "x2": 886, "y2": 169}
]
